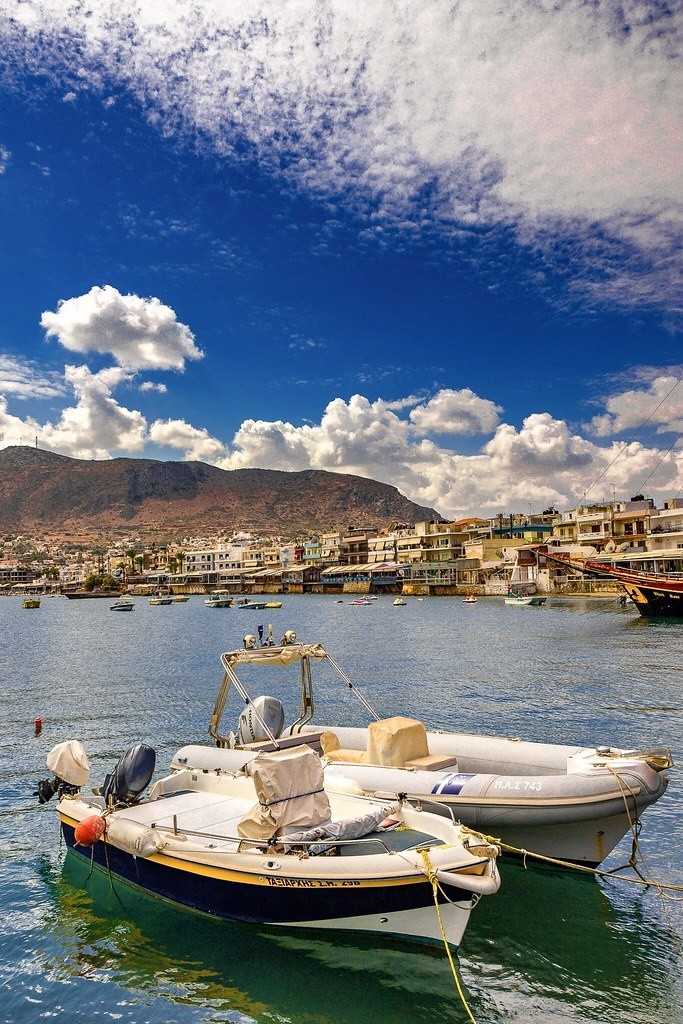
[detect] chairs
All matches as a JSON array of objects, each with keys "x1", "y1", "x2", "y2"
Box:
[{"x1": 237, "y1": 743, "x2": 332, "y2": 850}]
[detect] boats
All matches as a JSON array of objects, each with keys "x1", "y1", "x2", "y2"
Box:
[
  {"x1": 503, "y1": 586, "x2": 548, "y2": 606},
  {"x1": 205, "y1": 590, "x2": 233, "y2": 608},
  {"x1": 166, "y1": 595, "x2": 191, "y2": 602},
  {"x1": 109, "y1": 601, "x2": 135, "y2": 611},
  {"x1": 33, "y1": 739, "x2": 500, "y2": 955},
  {"x1": 148, "y1": 589, "x2": 174, "y2": 605},
  {"x1": 528, "y1": 376, "x2": 683, "y2": 623},
  {"x1": 393, "y1": 597, "x2": 407, "y2": 606},
  {"x1": 21, "y1": 596, "x2": 41, "y2": 608},
  {"x1": 359, "y1": 594, "x2": 378, "y2": 600},
  {"x1": 233, "y1": 595, "x2": 251, "y2": 605},
  {"x1": 462, "y1": 595, "x2": 478, "y2": 603},
  {"x1": 238, "y1": 602, "x2": 268, "y2": 610},
  {"x1": 264, "y1": 601, "x2": 283, "y2": 608},
  {"x1": 205, "y1": 622, "x2": 675, "y2": 874},
  {"x1": 116, "y1": 594, "x2": 136, "y2": 603},
  {"x1": 349, "y1": 599, "x2": 373, "y2": 605}
]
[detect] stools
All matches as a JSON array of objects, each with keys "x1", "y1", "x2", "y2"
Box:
[{"x1": 403, "y1": 754, "x2": 460, "y2": 774}]
